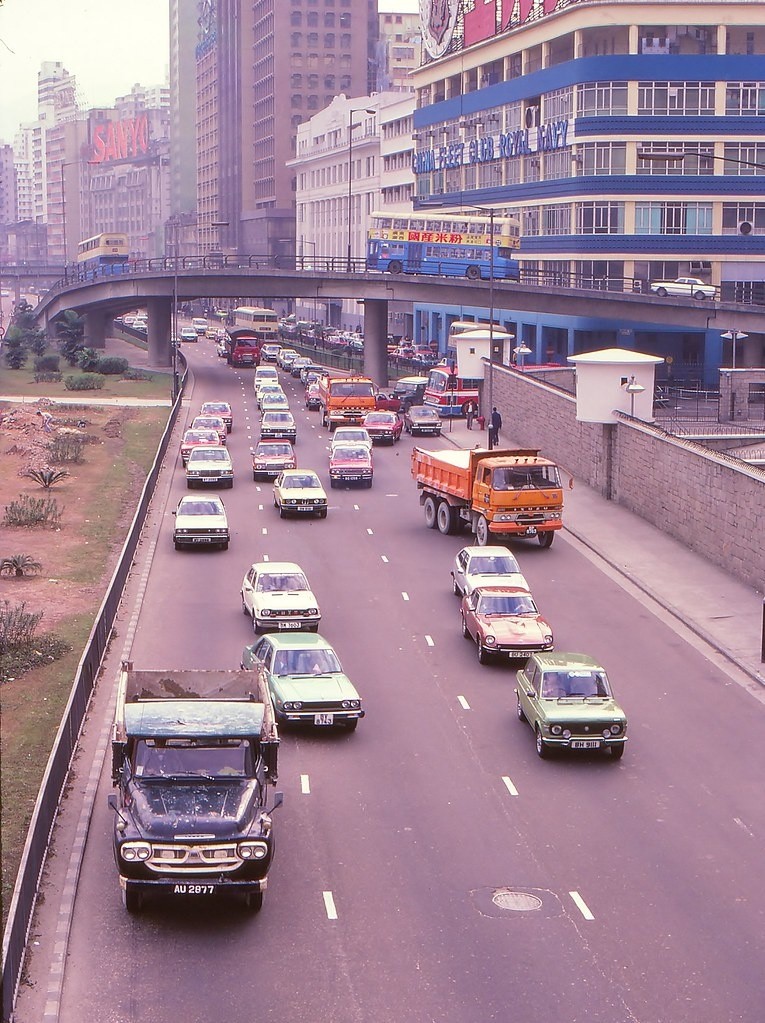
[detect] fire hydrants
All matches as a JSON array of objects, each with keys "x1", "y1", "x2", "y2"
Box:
[{"x1": 476, "y1": 417, "x2": 485, "y2": 430}]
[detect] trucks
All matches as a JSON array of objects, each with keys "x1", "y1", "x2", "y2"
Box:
[
  {"x1": 107, "y1": 661, "x2": 284, "y2": 915},
  {"x1": 317, "y1": 372, "x2": 379, "y2": 433},
  {"x1": 411, "y1": 445, "x2": 573, "y2": 547}
]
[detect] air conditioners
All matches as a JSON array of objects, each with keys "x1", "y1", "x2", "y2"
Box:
[
  {"x1": 571, "y1": 154, "x2": 581, "y2": 161},
  {"x1": 531, "y1": 160, "x2": 539, "y2": 167},
  {"x1": 460, "y1": 114, "x2": 499, "y2": 128},
  {"x1": 493, "y1": 166, "x2": 499, "y2": 172},
  {"x1": 412, "y1": 127, "x2": 448, "y2": 140}
]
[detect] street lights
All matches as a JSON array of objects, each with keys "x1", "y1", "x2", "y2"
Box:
[
  {"x1": 173, "y1": 222, "x2": 229, "y2": 397},
  {"x1": 440, "y1": 202, "x2": 494, "y2": 450},
  {"x1": 720, "y1": 330, "x2": 748, "y2": 370},
  {"x1": 61, "y1": 161, "x2": 100, "y2": 285},
  {"x1": 278, "y1": 239, "x2": 317, "y2": 344},
  {"x1": 347, "y1": 109, "x2": 374, "y2": 271}
]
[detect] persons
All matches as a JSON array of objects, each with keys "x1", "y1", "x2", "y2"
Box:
[
  {"x1": 263, "y1": 576, "x2": 275, "y2": 589},
  {"x1": 466, "y1": 400, "x2": 475, "y2": 430},
  {"x1": 481, "y1": 599, "x2": 496, "y2": 612},
  {"x1": 37, "y1": 412, "x2": 52, "y2": 433},
  {"x1": 543, "y1": 673, "x2": 565, "y2": 696},
  {"x1": 492, "y1": 407, "x2": 501, "y2": 446},
  {"x1": 275, "y1": 651, "x2": 293, "y2": 674},
  {"x1": 143, "y1": 737, "x2": 185, "y2": 774}
]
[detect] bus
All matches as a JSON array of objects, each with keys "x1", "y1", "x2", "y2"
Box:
[
  {"x1": 77, "y1": 232, "x2": 129, "y2": 282},
  {"x1": 423, "y1": 367, "x2": 561, "y2": 418},
  {"x1": 366, "y1": 211, "x2": 521, "y2": 281}
]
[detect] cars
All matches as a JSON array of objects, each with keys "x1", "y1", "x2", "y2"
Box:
[
  {"x1": 650, "y1": 277, "x2": 717, "y2": 300},
  {"x1": 273, "y1": 469, "x2": 327, "y2": 518},
  {"x1": 180, "y1": 401, "x2": 232, "y2": 468},
  {"x1": 376, "y1": 377, "x2": 442, "y2": 437},
  {"x1": 459, "y1": 587, "x2": 554, "y2": 664},
  {"x1": 361, "y1": 412, "x2": 403, "y2": 445},
  {"x1": 514, "y1": 652, "x2": 628, "y2": 759},
  {"x1": 250, "y1": 438, "x2": 297, "y2": 482},
  {"x1": 450, "y1": 546, "x2": 531, "y2": 598},
  {"x1": 116, "y1": 310, "x2": 148, "y2": 333},
  {"x1": 240, "y1": 562, "x2": 321, "y2": 634},
  {"x1": 171, "y1": 306, "x2": 439, "y2": 388},
  {"x1": 171, "y1": 494, "x2": 231, "y2": 550},
  {"x1": 240, "y1": 632, "x2": 364, "y2": 732},
  {"x1": 185, "y1": 446, "x2": 234, "y2": 488},
  {"x1": 330, "y1": 426, "x2": 373, "y2": 487},
  {"x1": 253, "y1": 365, "x2": 297, "y2": 445}
]
